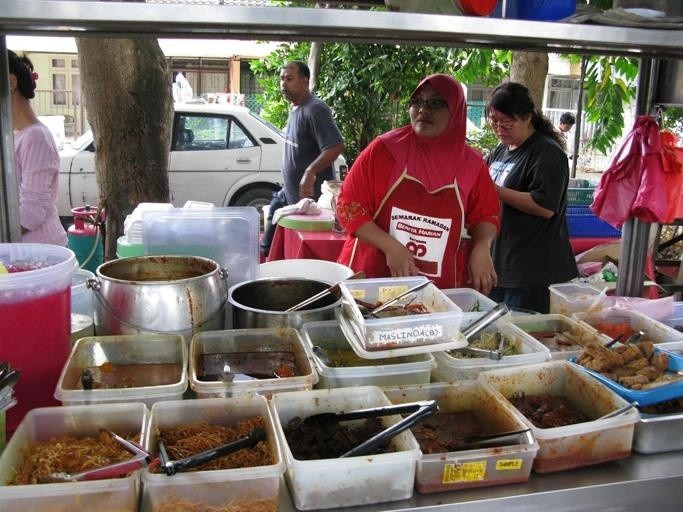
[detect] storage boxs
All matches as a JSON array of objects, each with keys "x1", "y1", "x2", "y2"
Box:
[
  {"x1": 300, "y1": 321, "x2": 437, "y2": 389},
  {"x1": 568, "y1": 188, "x2": 597, "y2": 206},
  {"x1": 441, "y1": 288, "x2": 498, "y2": 314},
  {"x1": 566, "y1": 208, "x2": 621, "y2": 238}
]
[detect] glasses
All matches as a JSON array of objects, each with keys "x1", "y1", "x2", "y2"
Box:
[
  {"x1": 410, "y1": 96, "x2": 447, "y2": 110},
  {"x1": 488, "y1": 118, "x2": 518, "y2": 130}
]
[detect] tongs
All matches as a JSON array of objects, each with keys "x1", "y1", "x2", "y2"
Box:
[
  {"x1": 40, "y1": 425, "x2": 152, "y2": 481},
  {"x1": 298, "y1": 399, "x2": 439, "y2": 458},
  {"x1": 444, "y1": 428, "x2": 536, "y2": 452},
  {"x1": 152, "y1": 422, "x2": 264, "y2": 477},
  {"x1": 458, "y1": 332, "x2": 506, "y2": 361}
]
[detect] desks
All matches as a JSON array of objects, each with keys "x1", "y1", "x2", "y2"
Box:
[
  {"x1": 277, "y1": 451, "x2": 683, "y2": 512},
  {"x1": 266, "y1": 224, "x2": 659, "y2": 299}
]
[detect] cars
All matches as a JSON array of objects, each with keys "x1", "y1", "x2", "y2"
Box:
[{"x1": 55, "y1": 99, "x2": 347, "y2": 230}]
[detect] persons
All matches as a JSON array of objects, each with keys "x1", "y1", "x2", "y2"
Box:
[
  {"x1": 5, "y1": 48, "x2": 70, "y2": 249},
  {"x1": 332, "y1": 74, "x2": 500, "y2": 294},
  {"x1": 259, "y1": 62, "x2": 345, "y2": 260},
  {"x1": 480, "y1": 83, "x2": 577, "y2": 314},
  {"x1": 556, "y1": 112, "x2": 577, "y2": 139}
]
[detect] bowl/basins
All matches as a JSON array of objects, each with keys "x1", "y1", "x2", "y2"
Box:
[
  {"x1": 437, "y1": 287, "x2": 510, "y2": 323},
  {"x1": 299, "y1": 319, "x2": 438, "y2": 401},
  {"x1": 566, "y1": 346, "x2": 682, "y2": 406},
  {"x1": 631, "y1": 414, "x2": 682, "y2": 454}
]
